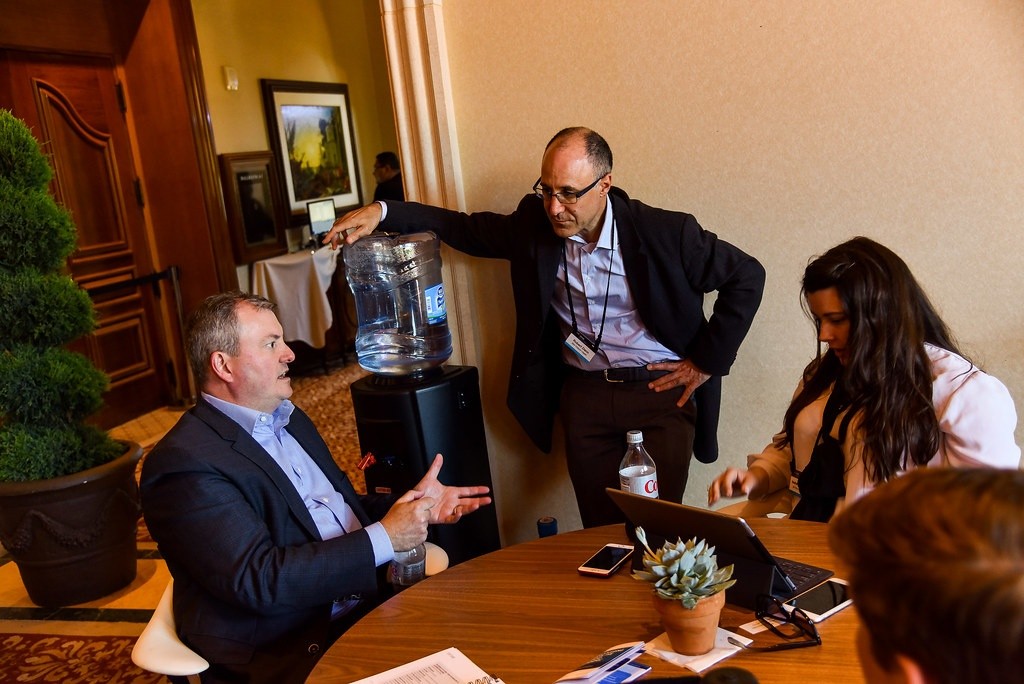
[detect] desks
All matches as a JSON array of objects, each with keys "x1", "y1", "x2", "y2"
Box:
[
  {"x1": 252, "y1": 244, "x2": 359, "y2": 377},
  {"x1": 303, "y1": 516, "x2": 863, "y2": 684}
]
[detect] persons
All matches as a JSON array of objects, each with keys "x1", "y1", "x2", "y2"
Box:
[
  {"x1": 237, "y1": 172, "x2": 275, "y2": 244},
  {"x1": 371, "y1": 151, "x2": 404, "y2": 204},
  {"x1": 708, "y1": 236, "x2": 1020, "y2": 523},
  {"x1": 320, "y1": 126, "x2": 766, "y2": 529},
  {"x1": 140, "y1": 293, "x2": 493, "y2": 684},
  {"x1": 825, "y1": 468, "x2": 1023, "y2": 683}
]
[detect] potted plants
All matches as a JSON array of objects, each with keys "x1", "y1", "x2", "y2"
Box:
[
  {"x1": 0, "y1": 106, "x2": 143, "y2": 608},
  {"x1": 630, "y1": 525, "x2": 737, "y2": 656}
]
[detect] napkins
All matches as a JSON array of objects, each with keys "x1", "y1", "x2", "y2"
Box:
[{"x1": 644, "y1": 626, "x2": 753, "y2": 674}]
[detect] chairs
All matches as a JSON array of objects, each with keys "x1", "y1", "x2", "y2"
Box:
[{"x1": 130, "y1": 542, "x2": 450, "y2": 684}]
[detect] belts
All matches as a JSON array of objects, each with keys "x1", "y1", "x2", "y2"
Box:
[{"x1": 564, "y1": 362, "x2": 673, "y2": 382}]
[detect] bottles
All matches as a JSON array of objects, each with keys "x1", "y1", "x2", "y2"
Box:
[
  {"x1": 390, "y1": 544, "x2": 426, "y2": 596},
  {"x1": 619, "y1": 429, "x2": 659, "y2": 543},
  {"x1": 344, "y1": 231, "x2": 455, "y2": 378}
]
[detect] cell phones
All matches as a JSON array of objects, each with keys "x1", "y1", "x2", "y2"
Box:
[
  {"x1": 578, "y1": 543, "x2": 634, "y2": 577},
  {"x1": 781, "y1": 576, "x2": 855, "y2": 623}
]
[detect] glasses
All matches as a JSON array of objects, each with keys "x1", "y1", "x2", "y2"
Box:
[
  {"x1": 532, "y1": 172, "x2": 609, "y2": 205},
  {"x1": 727, "y1": 597, "x2": 822, "y2": 652}
]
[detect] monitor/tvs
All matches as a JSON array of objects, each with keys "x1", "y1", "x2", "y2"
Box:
[{"x1": 306, "y1": 198, "x2": 336, "y2": 235}]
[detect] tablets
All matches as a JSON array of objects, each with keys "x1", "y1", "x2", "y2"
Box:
[{"x1": 606, "y1": 487, "x2": 797, "y2": 590}]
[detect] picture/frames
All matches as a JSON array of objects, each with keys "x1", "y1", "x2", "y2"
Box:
[
  {"x1": 218, "y1": 150, "x2": 289, "y2": 267},
  {"x1": 258, "y1": 76, "x2": 364, "y2": 231}
]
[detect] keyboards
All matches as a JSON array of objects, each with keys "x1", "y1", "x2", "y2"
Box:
[{"x1": 771, "y1": 556, "x2": 834, "y2": 599}]
